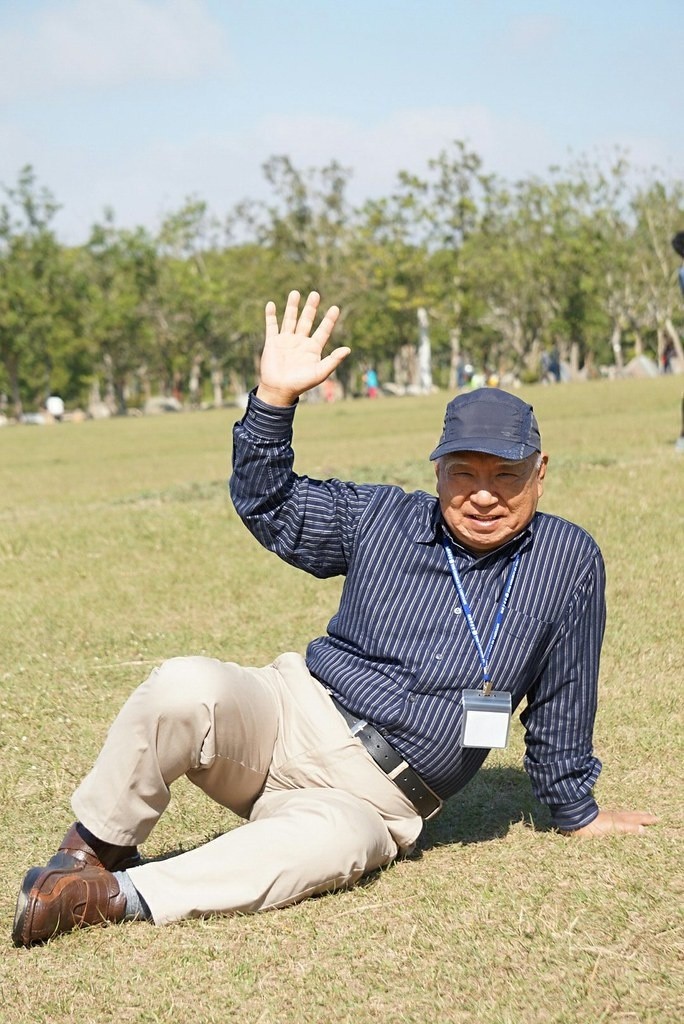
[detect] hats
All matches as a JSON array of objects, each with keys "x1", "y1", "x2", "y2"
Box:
[{"x1": 428, "y1": 387, "x2": 542, "y2": 462}]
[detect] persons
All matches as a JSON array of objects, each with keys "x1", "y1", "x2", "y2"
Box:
[
  {"x1": 0, "y1": 290, "x2": 676, "y2": 948},
  {"x1": 673, "y1": 230, "x2": 684, "y2": 450}
]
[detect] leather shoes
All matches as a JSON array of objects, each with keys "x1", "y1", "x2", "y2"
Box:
[
  {"x1": 10, "y1": 852, "x2": 127, "y2": 948},
  {"x1": 42, "y1": 822, "x2": 142, "y2": 875}
]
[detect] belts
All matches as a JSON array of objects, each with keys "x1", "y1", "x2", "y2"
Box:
[{"x1": 330, "y1": 690, "x2": 443, "y2": 821}]
